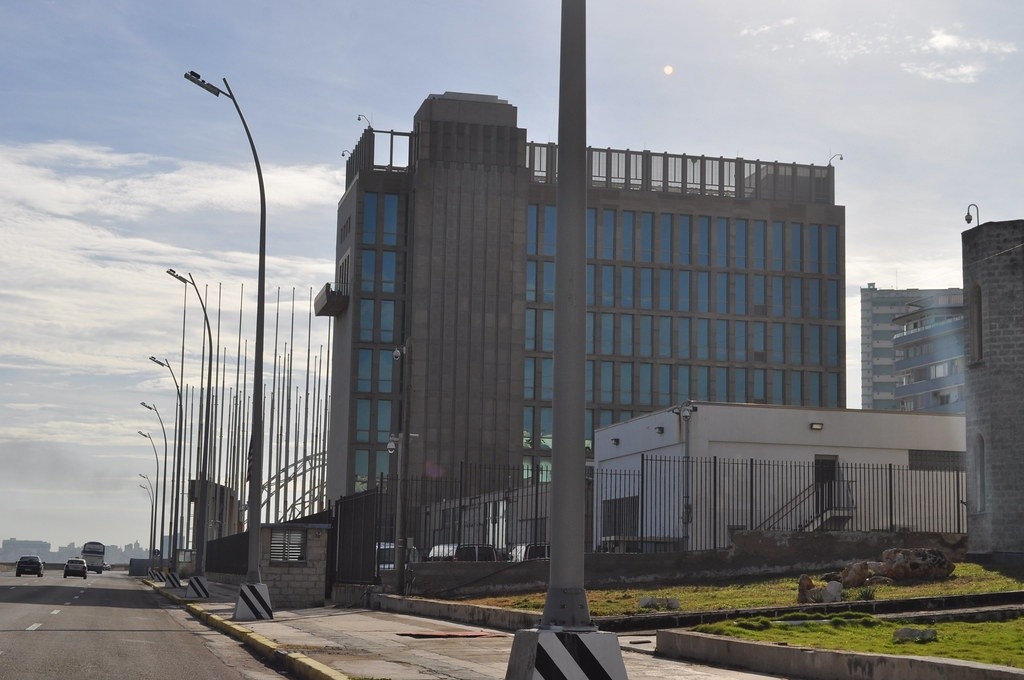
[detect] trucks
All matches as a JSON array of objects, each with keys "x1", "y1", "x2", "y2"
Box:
[
  {"x1": 375, "y1": 542, "x2": 421, "y2": 570},
  {"x1": 427, "y1": 542, "x2": 497, "y2": 561},
  {"x1": 508, "y1": 542, "x2": 551, "y2": 561}
]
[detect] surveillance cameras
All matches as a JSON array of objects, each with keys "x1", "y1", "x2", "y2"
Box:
[
  {"x1": 341, "y1": 150, "x2": 351, "y2": 157},
  {"x1": 358, "y1": 114, "x2": 370, "y2": 129},
  {"x1": 829, "y1": 154, "x2": 844, "y2": 167},
  {"x1": 386, "y1": 438, "x2": 398, "y2": 453},
  {"x1": 683, "y1": 410, "x2": 690, "y2": 421},
  {"x1": 964, "y1": 203, "x2": 980, "y2": 225},
  {"x1": 392, "y1": 345, "x2": 402, "y2": 360}
]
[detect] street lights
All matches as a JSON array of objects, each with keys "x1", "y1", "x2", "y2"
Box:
[
  {"x1": 384, "y1": 430, "x2": 419, "y2": 596},
  {"x1": 341, "y1": 149, "x2": 351, "y2": 157},
  {"x1": 358, "y1": 114, "x2": 371, "y2": 129},
  {"x1": 137, "y1": 430, "x2": 159, "y2": 582},
  {"x1": 140, "y1": 400, "x2": 167, "y2": 583},
  {"x1": 148, "y1": 354, "x2": 182, "y2": 589},
  {"x1": 166, "y1": 268, "x2": 211, "y2": 601},
  {"x1": 182, "y1": 66, "x2": 275, "y2": 622},
  {"x1": 385, "y1": 338, "x2": 420, "y2": 599}
]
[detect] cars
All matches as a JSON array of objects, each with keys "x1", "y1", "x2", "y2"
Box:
[
  {"x1": 103, "y1": 562, "x2": 112, "y2": 571},
  {"x1": 64, "y1": 557, "x2": 88, "y2": 580},
  {"x1": 16, "y1": 555, "x2": 46, "y2": 578}
]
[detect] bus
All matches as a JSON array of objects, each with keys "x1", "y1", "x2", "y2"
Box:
[{"x1": 81, "y1": 541, "x2": 106, "y2": 574}]
